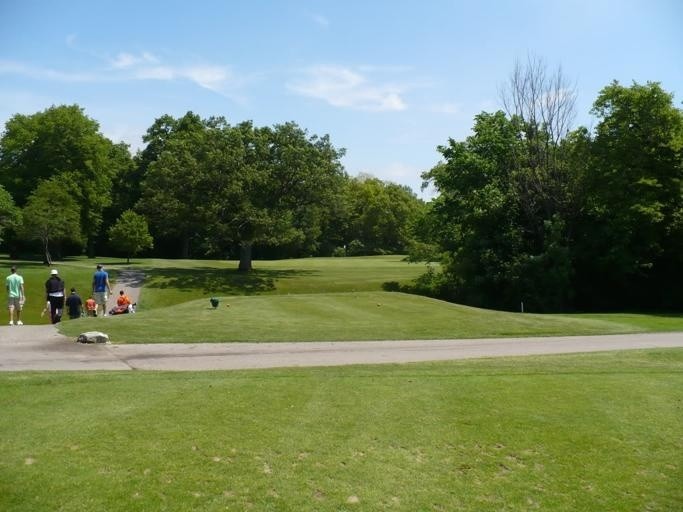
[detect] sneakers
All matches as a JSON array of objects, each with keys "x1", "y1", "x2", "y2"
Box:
[
  {"x1": 93, "y1": 310, "x2": 97, "y2": 316},
  {"x1": 17, "y1": 321, "x2": 24, "y2": 325},
  {"x1": 8, "y1": 321, "x2": 13, "y2": 325}
]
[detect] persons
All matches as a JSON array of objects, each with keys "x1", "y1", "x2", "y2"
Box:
[
  {"x1": 65, "y1": 287, "x2": 84, "y2": 320},
  {"x1": 44, "y1": 269, "x2": 65, "y2": 323},
  {"x1": 39, "y1": 298, "x2": 58, "y2": 324},
  {"x1": 4, "y1": 266, "x2": 24, "y2": 325},
  {"x1": 84, "y1": 297, "x2": 98, "y2": 316},
  {"x1": 117, "y1": 290, "x2": 135, "y2": 313},
  {"x1": 89, "y1": 264, "x2": 112, "y2": 316}
]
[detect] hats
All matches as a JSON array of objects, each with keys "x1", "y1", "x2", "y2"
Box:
[{"x1": 51, "y1": 270, "x2": 58, "y2": 275}]
